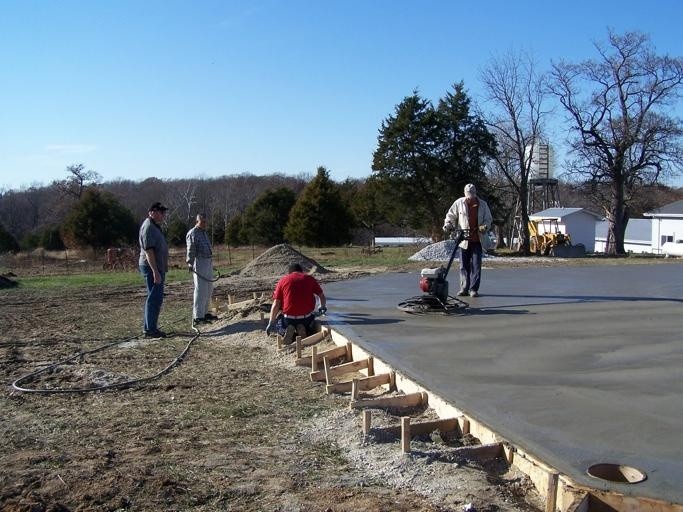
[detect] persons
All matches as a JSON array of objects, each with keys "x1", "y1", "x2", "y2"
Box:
[
  {"x1": 136, "y1": 201, "x2": 169, "y2": 337},
  {"x1": 265, "y1": 261, "x2": 327, "y2": 347},
  {"x1": 441, "y1": 183, "x2": 495, "y2": 297},
  {"x1": 556, "y1": 231, "x2": 571, "y2": 247},
  {"x1": 184, "y1": 212, "x2": 217, "y2": 325}
]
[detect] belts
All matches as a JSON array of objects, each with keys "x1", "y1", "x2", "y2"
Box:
[{"x1": 283, "y1": 313, "x2": 312, "y2": 319}]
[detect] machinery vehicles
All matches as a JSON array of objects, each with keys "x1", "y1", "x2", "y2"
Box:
[{"x1": 527, "y1": 217, "x2": 585, "y2": 257}]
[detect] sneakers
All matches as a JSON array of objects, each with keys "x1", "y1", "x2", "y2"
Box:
[
  {"x1": 283, "y1": 325, "x2": 295, "y2": 344},
  {"x1": 457, "y1": 289, "x2": 467, "y2": 295},
  {"x1": 144, "y1": 328, "x2": 164, "y2": 337},
  {"x1": 470, "y1": 291, "x2": 478, "y2": 297},
  {"x1": 297, "y1": 325, "x2": 307, "y2": 339},
  {"x1": 193, "y1": 314, "x2": 218, "y2": 324}
]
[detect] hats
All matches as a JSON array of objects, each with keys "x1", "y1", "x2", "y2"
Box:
[
  {"x1": 464, "y1": 184, "x2": 477, "y2": 198},
  {"x1": 149, "y1": 202, "x2": 169, "y2": 211}
]
